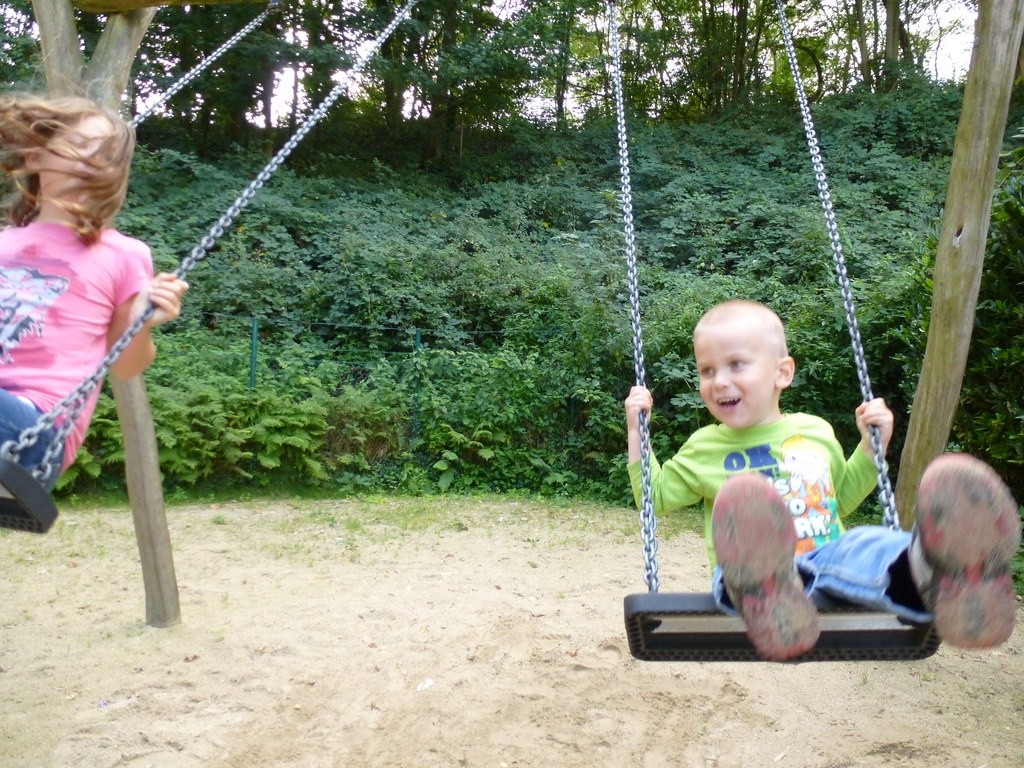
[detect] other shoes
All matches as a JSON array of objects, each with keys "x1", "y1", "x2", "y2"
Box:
[
  {"x1": 908, "y1": 454, "x2": 1021, "y2": 650},
  {"x1": 712, "y1": 473, "x2": 820, "y2": 659}
]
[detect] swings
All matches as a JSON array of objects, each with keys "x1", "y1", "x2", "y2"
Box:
[
  {"x1": 0, "y1": 2, "x2": 414, "y2": 533},
  {"x1": 607, "y1": 0, "x2": 945, "y2": 659}
]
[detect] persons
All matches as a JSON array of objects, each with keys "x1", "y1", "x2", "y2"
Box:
[
  {"x1": 0, "y1": 94, "x2": 190, "y2": 502},
  {"x1": 627, "y1": 301, "x2": 1021, "y2": 659}
]
